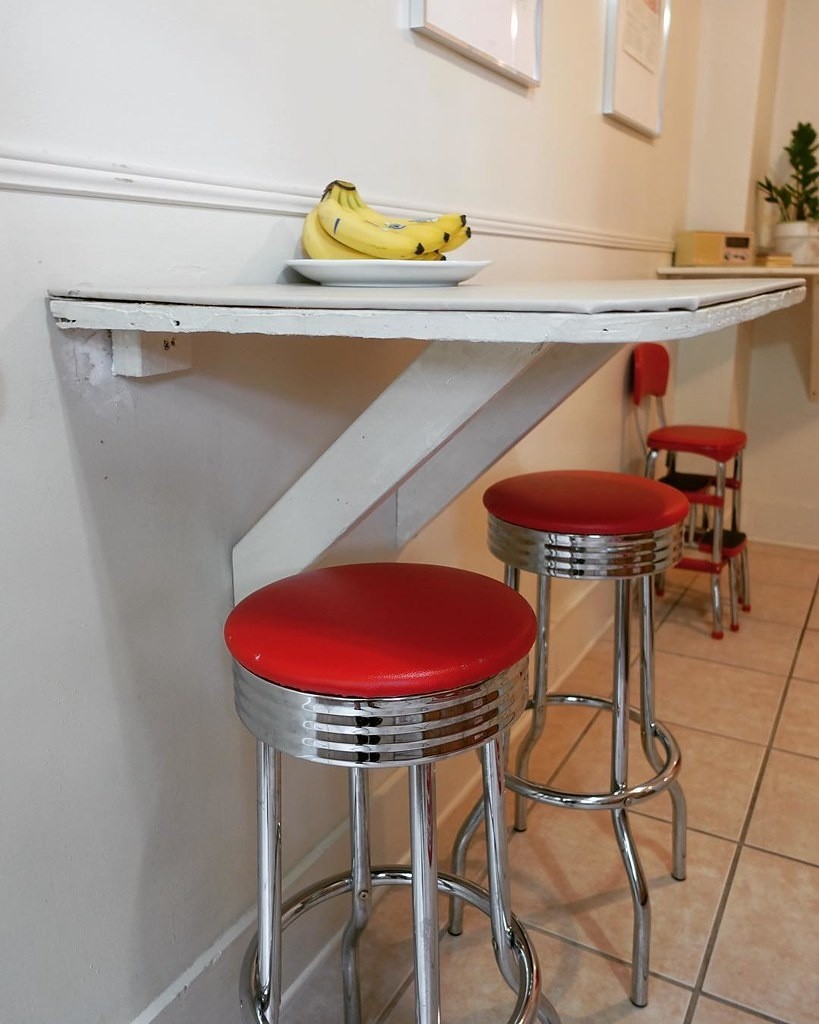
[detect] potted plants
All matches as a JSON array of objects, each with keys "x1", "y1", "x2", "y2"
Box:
[{"x1": 755, "y1": 121, "x2": 819, "y2": 266}]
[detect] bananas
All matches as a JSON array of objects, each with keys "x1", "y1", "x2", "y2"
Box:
[{"x1": 303, "y1": 180, "x2": 471, "y2": 259}]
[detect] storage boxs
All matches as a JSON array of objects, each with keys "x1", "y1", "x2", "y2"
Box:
[{"x1": 674, "y1": 231, "x2": 755, "y2": 267}]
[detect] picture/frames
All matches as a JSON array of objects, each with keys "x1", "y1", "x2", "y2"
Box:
[
  {"x1": 409, "y1": 0, "x2": 544, "y2": 89},
  {"x1": 600, "y1": 0, "x2": 672, "y2": 139}
]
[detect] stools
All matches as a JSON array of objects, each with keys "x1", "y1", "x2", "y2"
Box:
[
  {"x1": 627, "y1": 342, "x2": 752, "y2": 640},
  {"x1": 446, "y1": 469, "x2": 687, "y2": 1010},
  {"x1": 223, "y1": 559, "x2": 559, "y2": 1024}
]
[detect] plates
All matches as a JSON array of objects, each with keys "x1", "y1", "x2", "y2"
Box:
[{"x1": 284, "y1": 258, "x2": 493, "y2": 288}]
